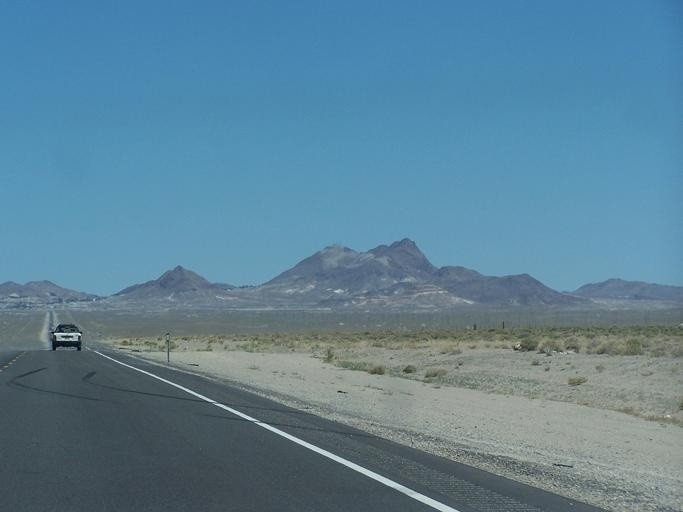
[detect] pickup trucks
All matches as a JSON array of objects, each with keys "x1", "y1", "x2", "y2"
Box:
[{"x1": 50, "y1": 324, "x2": 84, "y2": 352}]
